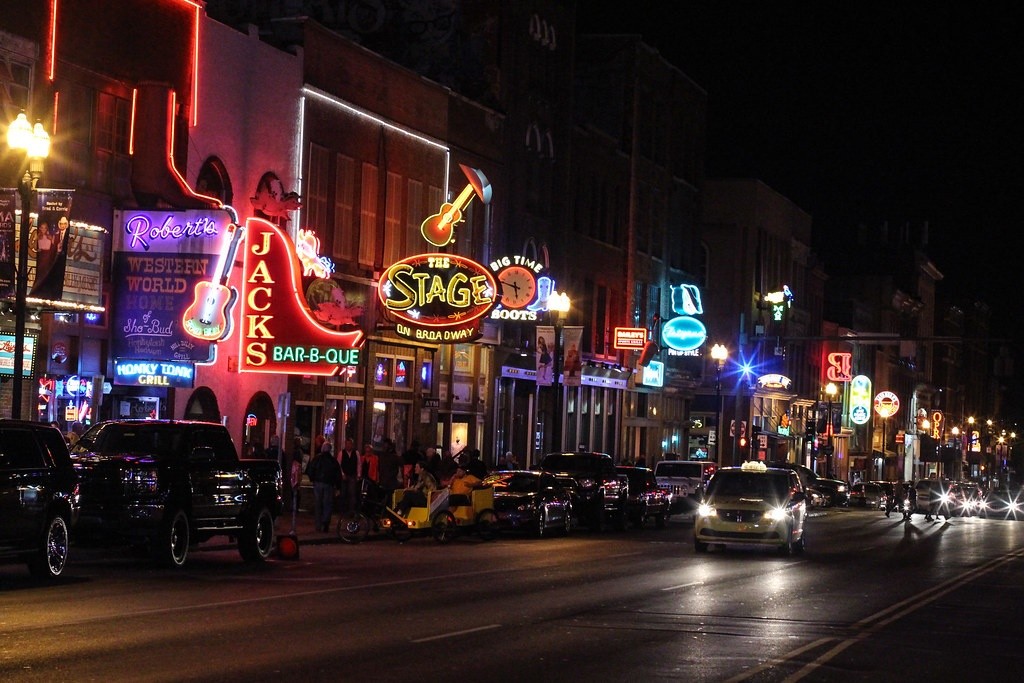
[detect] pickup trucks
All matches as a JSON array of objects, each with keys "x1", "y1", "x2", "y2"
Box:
[
  {"x1": 69, "y1": 418, "x2": 284, "y2": 570},
  {"x1": 530, "y1": 453, "x2": 629, "y2": 532}
]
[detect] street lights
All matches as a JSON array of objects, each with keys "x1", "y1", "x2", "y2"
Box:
[
  {"x1": 6, "y1": 112, "x2": 50, "y2": 420},
  {"x1": 710, "y1": 344, "x2": 728, "y2": 464},
  {"x1": 825, "y1": 383, "x2": 838, "y2": 479},
  {"x1": 548, "y1": 291, "x2": 570, "y2": 453}
]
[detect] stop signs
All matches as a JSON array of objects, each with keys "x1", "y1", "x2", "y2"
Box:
[{"x1": 730, "y1": 420, "x2": 746, "y2": 437}]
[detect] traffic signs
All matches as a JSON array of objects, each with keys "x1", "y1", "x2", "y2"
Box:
[{"x1": 708, "y1": 431, "x2": 716, "y2": 445}]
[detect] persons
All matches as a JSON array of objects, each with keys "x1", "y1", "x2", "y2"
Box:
[
  {"x1": 249, "y1": 434, "x2": 519, "y2": 533},
  {"x1": 885, "y1": 476, "x2": 951, "y2": 522},
  {"x1": 52, "y1": 421, "x2": 92, "y2": 450},
  {"x1": 536, "y1": 336, "x2": 552, "y2": 382},
  {"x1": 635, "y1": 455, "x2": 647, "y2": 468},
  {"x1": 30, "y1": 217, "x2": 69, "y2": 298}
]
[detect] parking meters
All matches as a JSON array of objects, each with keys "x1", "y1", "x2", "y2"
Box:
[{"x1": 290, "y1": 463, "x2": 303, "y2": 535}]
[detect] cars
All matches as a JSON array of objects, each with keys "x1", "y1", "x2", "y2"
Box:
[
  {"x1": 694, "y1": 467, "x2": 807, "y2": 556},
  {"x1": 654, "y1": 461, "x2": 712, "y2": 515},
  {"x1": 0, "y1": 416, "x2": 83, "y2": 583},
  {"x1": 615, "y1": 465, "x2": 673, "y2": 530},
  {"x1": 764, "y1": 461, "x2": 851, "y2": 509},
  {"x1": 912, "y1": 480, "x2": 1024, "y2": 522},
  {"x1": 481, "y1": 469, "x2": 573, "y2": 539},
  {"x1": 850, "y1": 481, "x2": 911, "y2": 510}
]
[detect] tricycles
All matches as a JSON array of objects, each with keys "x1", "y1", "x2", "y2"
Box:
[{"x1": 337, "y1": 478, "x2": 502, "y2": 545}]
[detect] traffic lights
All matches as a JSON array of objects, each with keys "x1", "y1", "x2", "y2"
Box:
[{"x1": 740, "y1": 439, "x2": 746, "y2": 446}]
